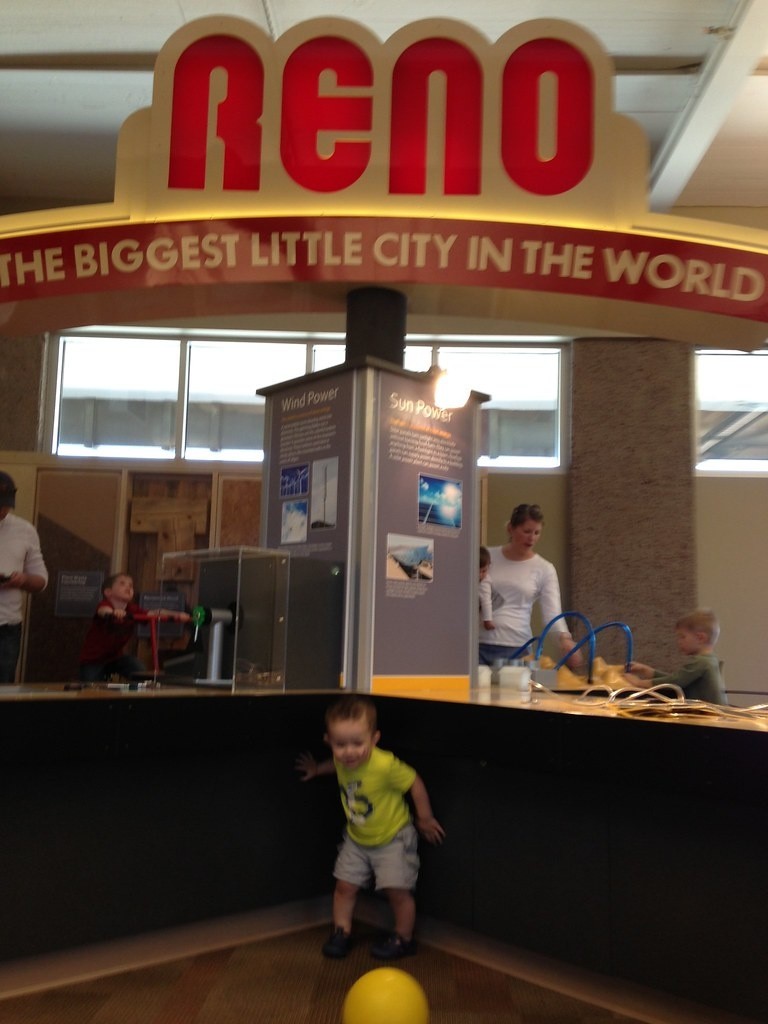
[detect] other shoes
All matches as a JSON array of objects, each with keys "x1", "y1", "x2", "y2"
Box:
[
  {"x1": 322, "y1": 927, "x2": 354, "y2": 959},
  {"x1": 370, "y1": 932, "x2": 419, "y2": 961}
]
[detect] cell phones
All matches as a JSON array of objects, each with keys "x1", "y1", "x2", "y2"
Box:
[{"x1": 0, "y1": 574, "x2": 10, "y2": 583}]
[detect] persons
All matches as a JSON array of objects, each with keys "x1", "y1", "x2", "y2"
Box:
[
  {"x1": 479, "y1": 503, "x2": 584, "y2": 667},
  {"x1": 76, "y1": 572, "x2": 190, "y2": 681},
  {"x1": 293, "y1": 698, "x2": 446, "y2": 959},
  {"x1": 479, "y1": 544, "x2": 495, "y2": 631},
  {"x1": 622, "y1": 608, "x2": 728, "y2": 706},
  {"x1": 1, "y1": 469, "x2": 49, "y2": 685}
]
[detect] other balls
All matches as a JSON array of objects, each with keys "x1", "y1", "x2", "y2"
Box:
[{"x1": 341, "y1": 965, "x2": 429, "y2": 1024}]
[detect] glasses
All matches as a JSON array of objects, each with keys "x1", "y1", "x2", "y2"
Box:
[{"x1": 513, "y1": 504, "x2": 541, "y2": 515}]
